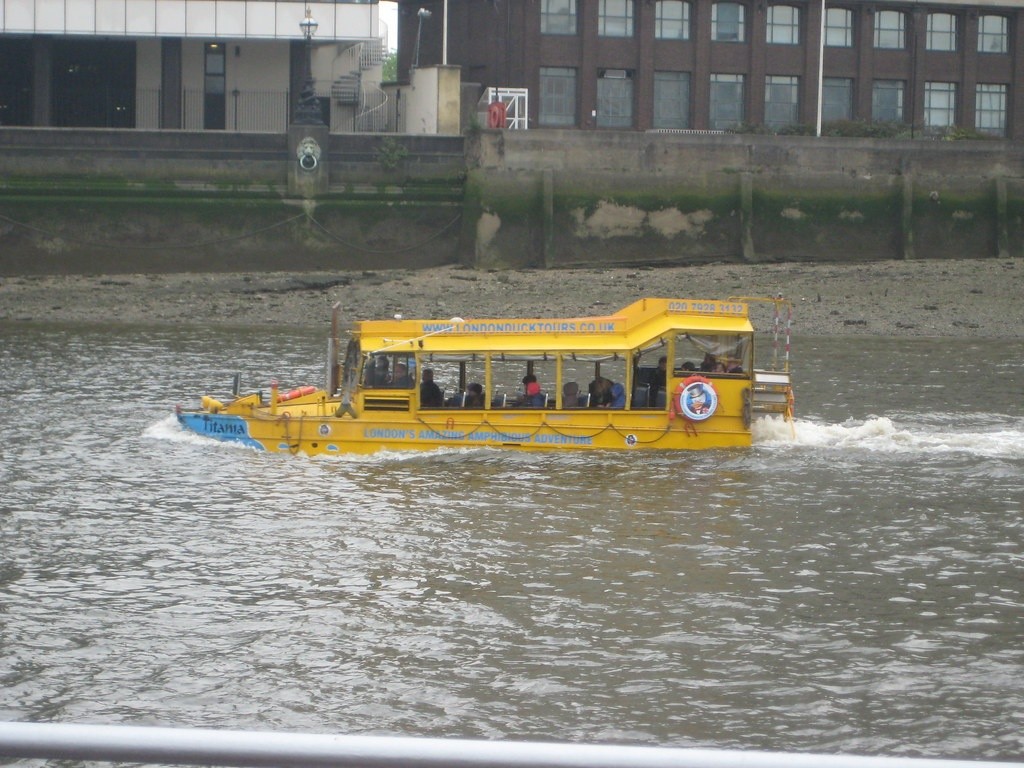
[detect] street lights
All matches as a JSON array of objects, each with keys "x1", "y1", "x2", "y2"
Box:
[{"x1": 293, "y1": 7, "x2": 324, "y2": 125}]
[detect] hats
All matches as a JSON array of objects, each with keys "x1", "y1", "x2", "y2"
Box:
[{"x1": 527, "y1": 382, "x2": 540, "y2": 396}]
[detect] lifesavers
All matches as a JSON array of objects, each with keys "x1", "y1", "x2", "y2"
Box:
[
  {"x1": 674, "y1": 376, "x2": 718, "y2": 422},
  {"x1": 490, "y1": 105, "x2": 500, "y2": 128},
  {"x1": 278, "y1": 386, "x2": 316, "y2": 402}
]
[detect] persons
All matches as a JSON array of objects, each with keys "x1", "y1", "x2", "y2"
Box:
[
  {"x1": 420, "y1": 368, "x2": 442, "y2": 408},
  {"x1": 588, "y1": 375, "x2": 626, "y2": 409},
  {"x1": 366, "y1": 356, "x2": 389, "y2": 385},
  {"x1": 384, "y1": 362, "x2": 414, "y2": 389},
  {"x1": 701, "y1": 355, "x2": 744, "y2": 374},
  {"x1": 681, "y1": 361, "x2": 695, "y2": 371},
  {"x1": 465, "y1": 383, "x2": 482, "y2": 410},
  {"x1": 515, "y1": 374, "x2": 543, "y2": 408},
  {"x1": 650, "y1": 354, "x2": 668, "y2": 408}
]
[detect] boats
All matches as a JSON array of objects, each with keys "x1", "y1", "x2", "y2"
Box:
[{"x1": 175, "y1": 295, "x2": 793, "y2": 454}]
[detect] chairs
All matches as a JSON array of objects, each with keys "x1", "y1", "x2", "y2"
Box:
[{"x1": 441, "y1": 382, "x2": 667, "y2": 411}]
